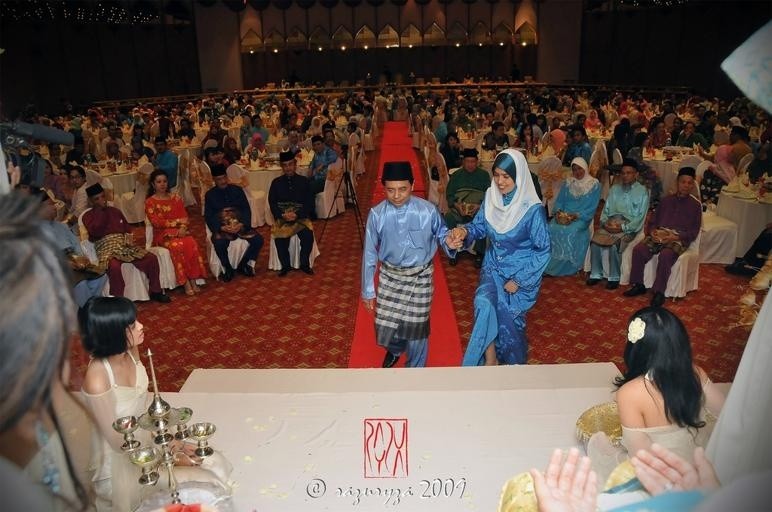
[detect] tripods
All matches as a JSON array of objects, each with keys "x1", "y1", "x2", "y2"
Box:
[{"x1": 318, "y1": 171, "x2": 364, "y2": 250}]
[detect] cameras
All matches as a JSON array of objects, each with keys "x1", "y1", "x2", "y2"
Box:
[{"x1": 340, "y1": 144, "x2": 349, "y2": 155}]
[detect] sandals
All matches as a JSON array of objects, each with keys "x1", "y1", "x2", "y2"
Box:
[{"x1": 184, "y1": 281, "x2": 201, "y2": 296}]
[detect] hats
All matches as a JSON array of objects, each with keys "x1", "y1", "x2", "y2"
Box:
[
  {"x1": 311, "y1": 135, "x2": 323, "y2": 145},
  {"x1": 155, "y1": 136, "x2": 167, "y2": 144},
  {"x1": 381, "y1": 162, "x2": 413, "y2": 183},
  {"x1": 679, "y1": 167, "x2": 695, "y2": 177},
  {"x1": 464, "y1": 148, "x2": 477, "y2": 157},
  {"x1": 85, "y1": 183, "x2": 103, "y2": 197},
  {"x1": 211, "y1": 163, "x2": 226, "y2": 177},
  {"x1": 623, "y1": 158, "x2": 638, "y2": 168},
  {"x1": 280, "y1": 151, "x2": 293, "y2": 162}
]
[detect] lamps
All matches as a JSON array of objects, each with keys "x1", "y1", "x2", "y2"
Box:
[{"x1": 112, "y1": 347, "x2": 216, "y2": 504}]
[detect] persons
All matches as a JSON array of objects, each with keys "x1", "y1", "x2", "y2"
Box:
[
  {"x1": 0, "y1": 74, "x2": 772, "y2": 304},
  {"x1": 0, "y1": 183, "x2": 106, "y2": 512},
  {"x1": 500, "y1": 441, "x2": 772, "y2": 512},
  {"x1": 77, "y1": 294, "x2": 236, "y2": 512},
  {"x1": 447, "y1": 146, "x2": 554, "y2": 365},
  {"x1": 358, "y1": 161, "x2": 463, "y2": 369},
  {"x1": 589, "y1": 304, "x2": 723, "y2": 458}
]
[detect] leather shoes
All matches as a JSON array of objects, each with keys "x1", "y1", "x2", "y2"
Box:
[
  {"x1": 224, "y1": 268, "x2": 234, "y2": 281},
  {"x1": 152, "y1": 292, "x2": 170, "y2": 303},
  {"x1": 300, "y1": 264, "x2": 313, "y2": 275},
  {"x1": 587, "y1": 279, "x2": 665, "y2": 306},
  {"x1": 278, "y1": 268, "x2": 290, "y2": 277},
  {"x1": 475, "y1": 257, "x2": 481, "y2": 267},
  {"x1": 382, "y1": 351, "x2": 399, "y2": 368},
  {"x1": 449, "y1": 259, "x2": 456, "y2": 265},
  {"x1": 238, "y1": 265, "x2": 253, "y2": 277}
]
